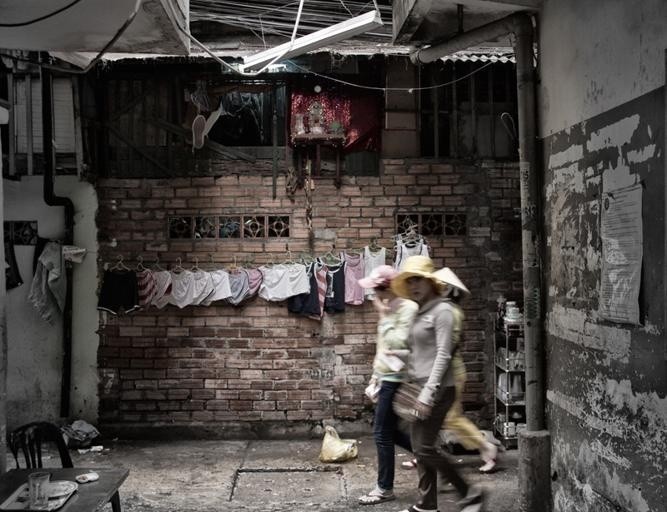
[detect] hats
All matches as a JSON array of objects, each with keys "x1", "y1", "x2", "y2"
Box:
[
  {"x1": 391, "y1": 256, "x2": 447, "y2": 298},
  {"x1": 358, "y1": 265, "x2": 398, "y2": 288},
  {"x1": 433, "y1": 267, "x2": 472, "y2": 299}
]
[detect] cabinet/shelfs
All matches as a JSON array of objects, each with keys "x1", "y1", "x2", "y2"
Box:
[{"x1": 492, "y1": 295, "x2": 526, "y2": 451}]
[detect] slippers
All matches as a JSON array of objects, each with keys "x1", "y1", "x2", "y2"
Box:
[
  {"x1": 401, "y1": 456, "x2": 417, "y2": 469},
  {"x1": 489, "y1": 460, "x2": 494, "y2": 464},
  {"x1": 358, "y1": 491, "x2": 395, "y2": 505},
  {"x1": 455, "y1": 487, "x2": 483, "y2": 512},
  {"x1": 401, "y1": 504, "x2": 440, "y2": 512}
]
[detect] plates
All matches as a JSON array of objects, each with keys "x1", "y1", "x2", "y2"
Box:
[{"x1": 19, "y1": 480, "x2": 78, "y2": 499}]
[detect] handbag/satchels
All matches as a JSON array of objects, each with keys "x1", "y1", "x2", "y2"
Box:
[{"x1": 392, "y1": 380, "x2": 429, "y2": 424}]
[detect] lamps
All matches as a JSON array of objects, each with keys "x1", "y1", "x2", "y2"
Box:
[{"x1": 243, "y1": 0, "x2": 385, "y2": 73}]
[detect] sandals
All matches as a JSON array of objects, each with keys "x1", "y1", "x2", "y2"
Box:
[{"x1": 479, "y1": 446, "x2": 498, "y2": 473}]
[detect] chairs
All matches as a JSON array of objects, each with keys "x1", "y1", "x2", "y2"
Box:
[{"x1": 9, "y1": 421, "x2": 76, "y2": 469}]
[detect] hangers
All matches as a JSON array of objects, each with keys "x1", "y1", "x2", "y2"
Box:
[{"x1": 106, "y1": 222, "x2": 433, "y2": 275}]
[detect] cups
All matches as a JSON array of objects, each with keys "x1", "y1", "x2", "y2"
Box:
[{"x1": 29, "y1": 472, "x2": 48, "y2": 511}]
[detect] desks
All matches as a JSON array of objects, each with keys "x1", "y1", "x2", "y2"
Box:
[{"x1": 0, "y1": 468, "x2": 130, "y2": 512}]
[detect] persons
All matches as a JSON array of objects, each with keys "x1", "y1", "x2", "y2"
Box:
[
  {"x1": 400, "y1": 264, "x2": 499, "y2": 474},
  {"x1": 391, "y1": 255, "x2": 485, "y2": 512},
  {"x1": 356, "y1": 265, "x2": 480, "y2": 505}
]
